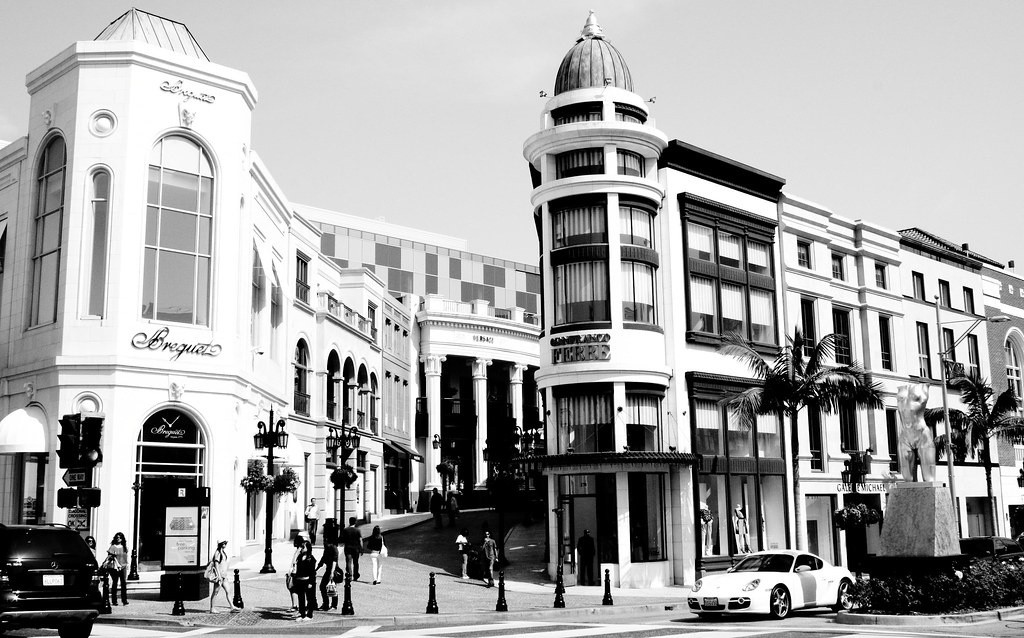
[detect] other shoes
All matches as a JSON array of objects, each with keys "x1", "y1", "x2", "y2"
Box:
[
  {"x1": 463, "y1": 576, "x2": 470, "y2": 579},
  {"x1": 113, "y1": 603, "x2": 118, "y2": 606},
  {"x1": 124, "y1": 601, "x2": 129, "y2": 605},
  {"x1": 328, "y1": 606, "x2": 338, "y2": 611},
  {"x1": 287, "y1": 607, "x2": 298, "y2": 612},
  {"x1": 292, "y1": 613, "x2": 301, "y2": 618},
  {"x1": 303, "y1": 616, "x2": 312, "y2": 620},
  {"x1": 349, "y1": 578, "x2": 352, "y2": 581},
  {"x1": 318, "y1": 605, "x2": 328, "y2": 611},
  {"x1": 486, "y1": 583, "x2": 494, "y2": 588},
  {"x1": 377, "y1": 582, "x2": 381, "y2": 584},
  {"x1": 296, "y1": 617, "x2": 303, "y2": 621},
  {"x1": 210, "y1": 610, "x2": 219, "y2": 614},
  {"x1": 232, "y1": 608, "x2": 241, "y2": 612},
  {"x1": 354, "y1": 574, "x2": 360, "y2": 581},
  {"x1": 373, "y1": 581, "x2": 376, "y2": 585}
]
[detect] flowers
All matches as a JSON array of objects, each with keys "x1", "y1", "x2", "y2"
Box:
[
  {"x1": 834, "y1": 501, "x2": 881, "y2": 528},
  {"x1": 241, "y1": 460, "x2": 302, "y2": 502}
]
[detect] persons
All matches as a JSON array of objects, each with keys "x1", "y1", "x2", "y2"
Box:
[
  {"x1": 455, "y1": 528, "x2": 474, "y2": 580},
  {"x1": 578, "y1": 529, "x2": 596, "y2": 586},
  {"x1": 897, "y1": 383, "x2": 936, "y2": 482},
  {"x1": 286, "y1": 531, "x2": 340, "y2": 621},
  {"x1": 343, "y1": 517, "x2": 364, "y2": 581},
  {"x1": 479, "y1": 531, "x2": 499, "y2": 588},
  {"x1": 430, "y1": 488, "x2": 444, "y2": 530},
  {"x1": 446, "y1": 492, "x2": 458, "y2": 529},
  {"x1": 367, "y1": 525, "x2": 386, "y2": 585},
  {"x1": 305, "y1": 498, "x2": 320, "y2": 545},
  {"x1": 213, "y1": 538, "x2": 241, "y2": 614},
  {"x1": 107, "y1": 532, "x2": 129, "y2": 606},
  {"x1": 85, "y1": 536, "x2": 96, "y2": 558},
  {"x1": 700, "y1": 505, "x2": 714, "y2": 556},
  {"x1": 734, "y1": 504, "x2": 753, "y2": 553}
]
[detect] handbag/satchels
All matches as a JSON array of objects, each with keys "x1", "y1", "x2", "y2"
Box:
[
  {"x1": 333, "y1": 567, "x2": 343, "y2": 583},
  {"x1": 204, "y1": 561, "x2": 219, "y2": 583},
  {"x1": 380, "y1": 536, "x2": 387, "y2": 558},
  {"x1": 326, "y1": 580, "x2": 338, "y2": 597}
]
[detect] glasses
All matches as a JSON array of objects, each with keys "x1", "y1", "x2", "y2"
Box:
[{"x1": 88, "y1": 540, "x2": 94, "y2": 543}]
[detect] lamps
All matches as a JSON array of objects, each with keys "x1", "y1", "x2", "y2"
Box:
[
  {"x1": 567, "y1": 447, "x2": 575, "y2": 453},
  {"x1": 714, "y1": 455, "x2": 726, "y2": 460},
  {"x1": 669, "y1": 445, "x2": 676, "y2": 452},
  {"x1": 644, "y1": 97, "x2": 656, "y2": 103},
  {"x1": 603, "y1": 78, "x2": 613, "y2": 87},
  {"x1": 865, "y1": 448, "x2": 874, "y2": 454},
  {"x1": 539, "y1": 91, "x2": 551, "y2": 98},
  {"x1": 623, "y1": 446, "x2": 630, "y2": 452}
]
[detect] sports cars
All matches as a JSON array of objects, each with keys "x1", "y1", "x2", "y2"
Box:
[{"x1": 686, "y1": 547, "x2": 858, "y2": 620}]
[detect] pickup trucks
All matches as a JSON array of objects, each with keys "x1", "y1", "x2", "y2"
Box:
[{"x1": 958, "y1": 537, "x2": 1024, "y2": 565}]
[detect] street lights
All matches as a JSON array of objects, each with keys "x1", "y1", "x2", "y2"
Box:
[
  {"x1": 483, "y1": 435, "x2": 511, "y2": 566},
  {"x1": 433, "y1": 433, "x2": 456, "y2": 502},
  {"x1": 936, "y1": 316, "x2": 1012, "y2": 537},
  {"x1": 513, "y1": 425, "x2": 541, "y2": 507},
  {"x1": 255, "y1": 421, "x2": 289, "y2": 572},
  {"x1": 327, "y1": 423, "x2": 362, "y2": 616}
]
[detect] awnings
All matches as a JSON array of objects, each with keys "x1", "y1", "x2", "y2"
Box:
[
  {"x1": 383, "y1": 440, "x2": 424, "y2": 463},
  {"x1": 273, "y1": 255, "x2": 294, "y2": 303},
  {"x1": 0, "y1": 406, "x2": 49, "y2": 454},
  {"x1": 253, "y1": 232, "x2": 278, "y2": 288}
]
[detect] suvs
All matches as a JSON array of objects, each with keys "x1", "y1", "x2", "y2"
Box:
[{"x1": 0, "y1": 521, "x2": 101, "y2": 638}]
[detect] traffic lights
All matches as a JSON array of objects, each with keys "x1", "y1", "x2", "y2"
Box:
[
  {"x1": 56, "y1": 413, "x2": 80, "y2": 469},
  {"x1": 82, "y1": 416, "x2": 102, "y2": 468}
]
